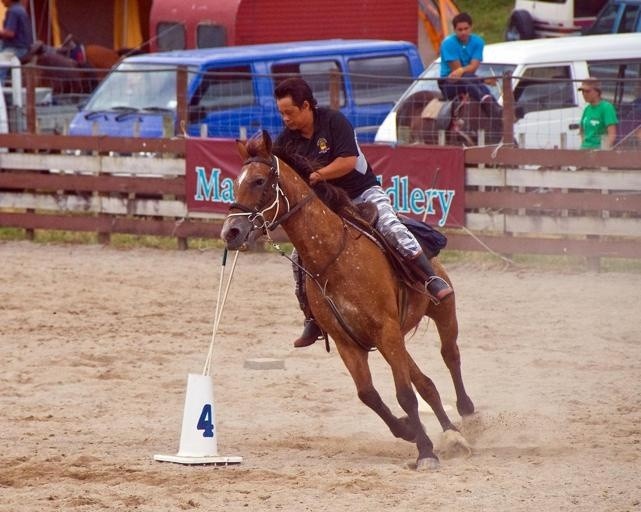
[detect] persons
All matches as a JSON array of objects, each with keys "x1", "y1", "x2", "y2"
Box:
[
  {"x1": 270, "y1": 77, "x2": 456, "y2": 350},
  {"x1": 437, "y1": 13, "x2": 526, "y2": 129},
  {"x1": 576, "y1": 77, "x2": 621, "y2": 221},
  {"x1": 1, "y1": 0, "x2": 37, "y2": 103}
]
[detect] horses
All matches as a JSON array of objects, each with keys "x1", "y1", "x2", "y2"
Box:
[
  {"x1": 396, "y1": 91, "x2": 519, "y2": 213},
  {"x1": 221, "y1": 128, "x2": 488, "y2": 472}
]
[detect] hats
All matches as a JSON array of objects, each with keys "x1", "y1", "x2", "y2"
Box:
[{"x1": 578, "y1": 77, "x2": 601, "y2": 91}]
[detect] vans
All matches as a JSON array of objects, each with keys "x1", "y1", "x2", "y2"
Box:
[
  {"x1": 372, "y1": 31, "x2": 641, "y2": 213},
  {"x1": 65, "y1": 38, "x2": 424, "y2": 219},
  {"x1": 148, "y1": 1, "x2": 462, "y2": 69}
]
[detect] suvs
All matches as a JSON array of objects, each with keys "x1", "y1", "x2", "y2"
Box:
[{"x1": 506, "y1": 0, "x2": 641, "y2": 43}]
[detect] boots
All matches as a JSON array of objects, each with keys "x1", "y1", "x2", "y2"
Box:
[
  {"x1": 409, "y1": 249, "x2": 453, "y2": 300},
  {"x1": 480, "y1": 97, "x2": 502, "y2": 127},
  {"x1": 293, "y1": 293, "x2": 322, "y2": 347}
]
